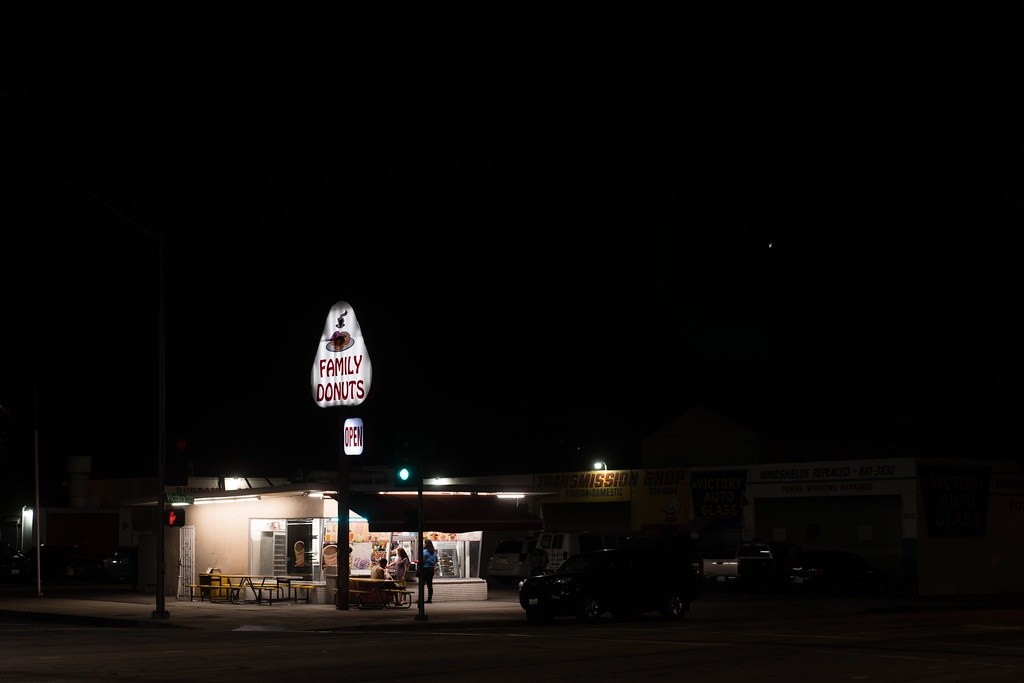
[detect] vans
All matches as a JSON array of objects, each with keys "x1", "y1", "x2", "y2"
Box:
[{"x1": 535, "y1": 529, "x2": 626, "y2": 574}]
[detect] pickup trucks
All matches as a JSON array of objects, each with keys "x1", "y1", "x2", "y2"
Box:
[{"x1": 701, "y1": 541, "x2": 802, "y2": 596}]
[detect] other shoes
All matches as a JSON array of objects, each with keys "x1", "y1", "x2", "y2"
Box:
[
  {"x1": 425, "y1": 600, "x2": 432, "y2": 602},
  {"x1": 401, "y1": 601, "x2": 407, "y2": 604}
]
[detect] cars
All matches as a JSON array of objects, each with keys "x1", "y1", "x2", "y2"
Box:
[
  {"x1": 102, "y1": 549, "x2": 136, "y2": 583},
  {"x1": 782, "y1": 550, "x2": 876, "y2": 599},
  {"x1": 0, "y1": 540, "x2": 33, "y2": 587},
  {"x1": 623, "y1": 534, "x2": 700, "y2": 579},
  {"x1": 25, "y1": 544, "x2": 99, "y2": 585}
]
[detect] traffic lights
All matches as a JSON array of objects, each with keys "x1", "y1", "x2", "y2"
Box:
[
  {"x1": 166, "y1": 509, "x2": 185, "y2": 526},
  {"x1": 164, "y1": 426, "x2": 189, "y2": 486},
  {"x1": 394, "y1": 452, "x2": 424, "y2": 488}
]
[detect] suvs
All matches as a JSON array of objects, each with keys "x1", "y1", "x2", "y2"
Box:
[
  {"x1": 517, "y1": 548, "x2": 699, "y2": 625},
  {"x1": 486, "y1": 536, "x2": 538, "y2": 583}
]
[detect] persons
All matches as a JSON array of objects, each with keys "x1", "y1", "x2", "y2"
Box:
[
  {"x1": 386, "y1": 548, "x2": 411, "y2": 604},
  {"x1": 414, "y1": 540, "x2": 438, "y2": 603},
  {"x1": 371, "y1": 558, "x2": 406, "y2": 606}
]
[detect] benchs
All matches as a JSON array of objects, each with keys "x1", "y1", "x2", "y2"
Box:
[
  {"x1": 370, "y1": 588, "x2": 415, "y2": 606},
  {"x1": 186, "y1": 583, "x2": 314, "y2": 606},
  {"x1": 332, "y1": 588, "x2": 371, "y2": 607}
]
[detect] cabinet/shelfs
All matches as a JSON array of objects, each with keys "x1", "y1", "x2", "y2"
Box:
[{"x1": 260, "y1": 530, "x2": 287, "y2": 576}]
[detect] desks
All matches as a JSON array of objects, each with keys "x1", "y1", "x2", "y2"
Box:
[
  {"x1": 350, "y1": 577, "x2": 402, "y2": 607},
  {"x1": 211, "y1": 574, "x2": 303, "y2": 604}
]
